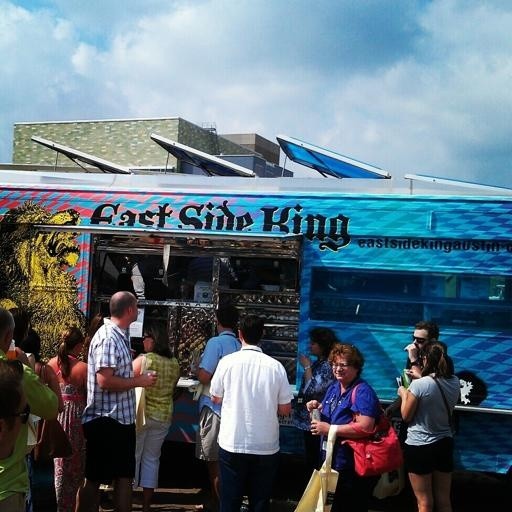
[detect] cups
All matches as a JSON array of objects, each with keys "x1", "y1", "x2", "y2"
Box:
[{"x1": 7, "y1": 339, "x2": 17, "y2": 359}]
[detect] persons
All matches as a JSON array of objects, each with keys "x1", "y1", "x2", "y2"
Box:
[
  {"x1": 386, "y1": 320, "x2": 462, "y2": 512},
  {"x1": 197, "y1": 304, "x2": 242, "y2": 512},
  {"x1": 286, "y1": 327, "x2": 339, "y2": 500},
  {"x1": 305, "y1": 344, "x2": 384, "y2": 511},
  {"x1": 209, "y1": 315, "x2": 294, "y2": 512},
  {"x1": 2, "y1": 308, "x2": 63, "y2": 512},
  {"x1": 46, "y1": 313, "x2": 179, "y2": 512},
  {"x1": 81, "y1": 290, "x2": 158, "y2": 512}
]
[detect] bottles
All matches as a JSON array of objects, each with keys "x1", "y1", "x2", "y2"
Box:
[{"x1": 311, "y1": 409, "x2": 321, "y2": 422}]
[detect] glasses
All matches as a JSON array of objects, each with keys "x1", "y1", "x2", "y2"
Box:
[
  {"x1": 14, "y1": 404, "x2": 30, "y2": 424},
  {"x1": 413, "y1": 336, "x2": 429, "y2": 344},
  {"x1": 332, "y1": 362, "x2": 352, "y2": 368},
  {"x1": 142, "y1": 335, "x2": 151, "y2": 340}
]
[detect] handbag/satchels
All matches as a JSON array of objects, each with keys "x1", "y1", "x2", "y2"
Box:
[
  {"x1": 135, "y1": 387, "x2": 145, "y2": 431},
  {"x1": 33, "y1": 419, "x2": 71, "y2": 462},
  {"x1": 294, "y1": 461, "x2": 339, "y2": 512},
  {"x1": 346, "y1": 423, "x2": 404, "y2": 477},
  {"x1": 373, "y1": 465, "x2": 404, "y2": 498}
]
